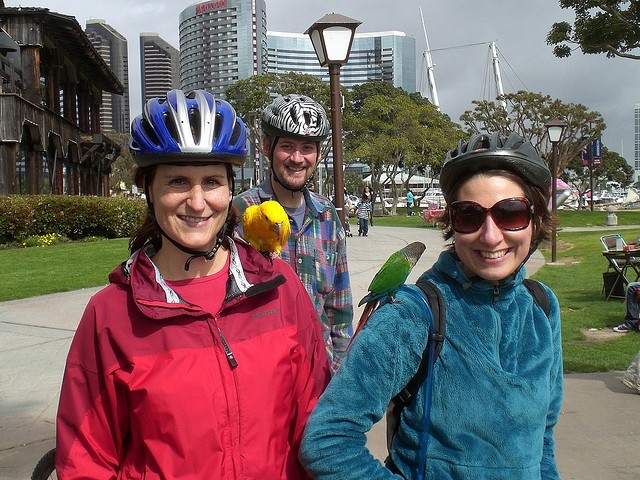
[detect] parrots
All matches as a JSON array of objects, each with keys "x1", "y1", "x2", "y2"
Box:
[
  {"x1": 344, "y1": 241, "x2": 426, "y2": 355},
  {"x1": 242, "y1": 201, "x2": 292, "y2": 257}
]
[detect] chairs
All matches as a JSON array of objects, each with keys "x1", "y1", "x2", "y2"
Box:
[{"x1": 599, "y1": 233, "x2": 640, "y2": 296}]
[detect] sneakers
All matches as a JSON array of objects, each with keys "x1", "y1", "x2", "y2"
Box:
[
  {"x1": 358, "y1": 233, "x2": 361, "y2": 236},
  {"x1": 362, "y1": 232, "x2": 368, "y2": 236},
  {"x1": 613, "y1": 323, "x2": 630, "y2": 332},
  {"x1": 621, "y1": 376, "x2": 640, "y2": 393}
]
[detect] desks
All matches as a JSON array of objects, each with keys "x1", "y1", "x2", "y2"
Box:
[{"x1": 601, "y1": 247, "x2": 640, "y2": 302}]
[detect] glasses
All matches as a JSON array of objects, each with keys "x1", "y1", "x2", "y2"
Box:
[{"x1": 447, "y1": 197, "x2": 537, "y2": 235}]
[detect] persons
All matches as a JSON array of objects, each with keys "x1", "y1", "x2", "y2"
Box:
[
  {"x1": 53, "y1": 87, "x2": 332, "y2": 480},
  {"x1": 609, "y1": 282, "x2": 640, "y2": 334},
  {"x1": 405, "y1": 187, "x2": 416, "y2": 218},
  {"x1": 359, "y1": 184, "x2": 375, "y2": 228},
  {"x1": 295, "y1": 123, "x2": 567, "y2": 479},
  {"x1": 226, "y1": 93, "x2": 356, "y2": 383},
  {"x1": 331, "y1": 186, "x2": 351, "y2": 236},
  {"x1": 353, "y1": 193, "x2": 371, "y2": 238},
  {"x1": 307, "y1": 182, "x2": 315, "y2": 193}
]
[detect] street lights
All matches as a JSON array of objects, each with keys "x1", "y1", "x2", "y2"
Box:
[
  {"x1": 303, "y1": 11, "x2": 361, "y2": 234},
  {"x1": 544, "y1": 120, "x2": 568, "y2": 263}
]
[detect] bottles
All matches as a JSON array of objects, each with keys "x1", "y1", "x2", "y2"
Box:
[{"x1": 615, "y1": 234, "x2": 622, "y2": 251}]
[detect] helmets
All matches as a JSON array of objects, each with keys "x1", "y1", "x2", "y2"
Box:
[
  {"x1": 128, "y1": 89, "x2": 247, "y2": 165},
  {"x1": 440, "y1": 131, "x2": 550, "y2": 207},
  {"x1": 261, "y1": 93, "x2": 330, "y2": 140}
]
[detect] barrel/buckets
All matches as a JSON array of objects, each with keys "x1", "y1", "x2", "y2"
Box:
[{"x1": 602, "y1": 263, "x2": 625, "y2": 300}]
